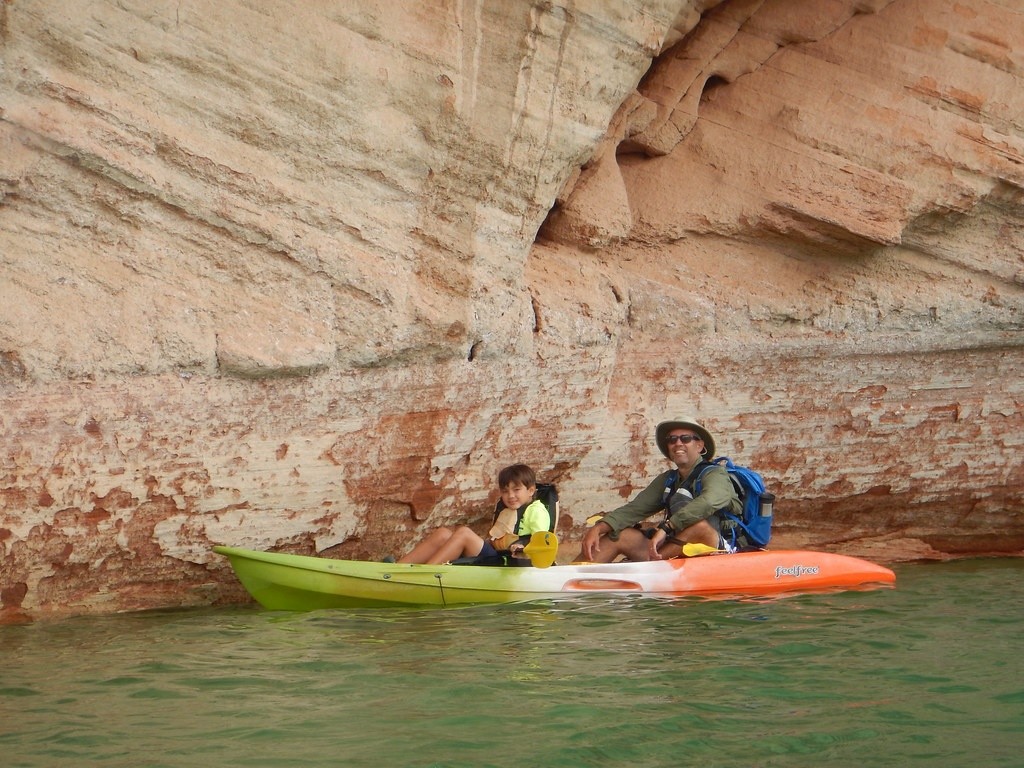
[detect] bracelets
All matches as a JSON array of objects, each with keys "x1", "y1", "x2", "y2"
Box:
[{"x1": 658, "y1": 521, "x2": 672, "y2": 534}]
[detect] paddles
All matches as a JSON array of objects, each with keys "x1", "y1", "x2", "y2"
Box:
[
  {"x1": 588, "y1": 515, "x2": 728, "y2": 558},
  {"x1": 498, "y1": 531, "x2": 559, "y2": 568}
]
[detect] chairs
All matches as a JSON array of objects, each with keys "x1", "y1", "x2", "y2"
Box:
[{"x1": 493, "y1": 485, "x2": 560, "y2": 567}]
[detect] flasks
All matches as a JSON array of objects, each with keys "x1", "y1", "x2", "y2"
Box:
[{"x1": 759, "y1": 493, "x2": 775, "y2": 517}]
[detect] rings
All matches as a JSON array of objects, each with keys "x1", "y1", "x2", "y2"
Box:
[{"x1": 650, "y1": 548, "x2": 652, "y2": 550}]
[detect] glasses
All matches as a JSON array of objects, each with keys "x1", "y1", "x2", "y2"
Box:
[{"x1": 664, "y1": 434, "x2": 699, "y2": 444}]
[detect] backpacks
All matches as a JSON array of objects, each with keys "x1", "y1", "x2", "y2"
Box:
[{"x1": 663, "y1": 457, "x2": 775, "y2": 550}]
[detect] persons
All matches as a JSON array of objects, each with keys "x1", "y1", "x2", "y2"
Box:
[
  {"x1": 572, "y1": 416, "x2": 747, "y2": 563},
  {"x1": 384, "y1": 465, "x2": 550, "y2": 564}
]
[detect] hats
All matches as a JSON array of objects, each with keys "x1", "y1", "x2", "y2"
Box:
[{"x1": 655, "y1": 416, "x2": 716, "y2": 461}]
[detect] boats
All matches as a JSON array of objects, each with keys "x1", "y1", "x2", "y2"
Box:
[{"x1": 212, "y1": 543, "x2": 897, "y2": 609}]
